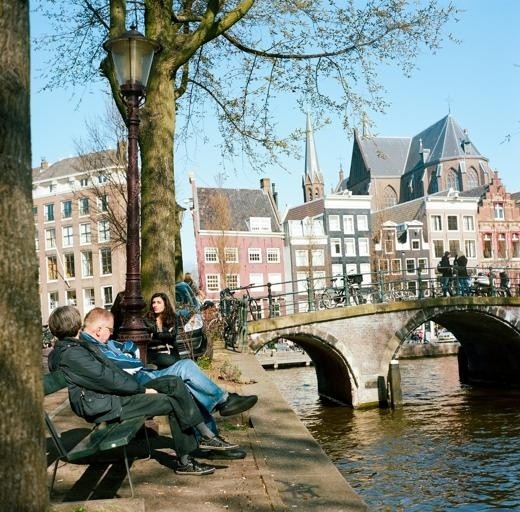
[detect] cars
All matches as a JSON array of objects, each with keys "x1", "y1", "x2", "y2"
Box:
[
  {"x1": 41, "y1": 324, "x2": 53, "y2": 349},
  {"x1": 170, "y1": 279, "x2": 216, "y2": 359},
  {"x1": 438, "y1": 327, "x2": 457, "y2": 342}
]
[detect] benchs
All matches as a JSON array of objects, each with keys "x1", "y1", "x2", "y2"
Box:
[
  {"x1": 172, "y1": 329, "x2": 208, "y2": 359},
  {"x1": 40, "y1": 371, "x2": 151, "y2": 502},
  {"x1": 478, "y1": 287, "x2": 505, "y2": 297}
]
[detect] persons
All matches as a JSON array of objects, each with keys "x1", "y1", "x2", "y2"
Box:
[
  {"x1": 453, "y1": 251, "x2": 471, "y2": 296},
  {"x1": 46, "y1": 305, "x2": 240, "y2": 476},
  {"x1": 142, "y1": 292, "x2": 183, "y2": 371},
  {"x1": 438, "y1": 251, "x2": 454, "y2": 297},
  {"x1": 500, "y1": 271, "x2": 512, "y2": 297},
  {"x1": 79, "y1": 307, "x2": 258, "y2": 460}
]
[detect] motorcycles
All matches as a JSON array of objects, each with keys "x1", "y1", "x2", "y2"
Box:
[
  {"x1": 474, "y1": 265, "x2": 512, "y2": 297},
  {"x1": 467, "y1": 276, "x2": 477, "y2": 294}
]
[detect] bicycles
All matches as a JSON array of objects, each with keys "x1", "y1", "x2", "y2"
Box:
[
  {"x1": 321, "y1": 269, "x2": 359, "y2": 309},
  {"x1": 206, "y1": 317, "x2": 229, "y2": 331},
  {"x1": 228, "y1": 283, "x2": 260, "y2": 322},
  {"x1": 370, "y1": 279, "x2": 416, "y2": 303},
  {"x1": 223, "y1": 299, "x2": 247, "y2": 353}
]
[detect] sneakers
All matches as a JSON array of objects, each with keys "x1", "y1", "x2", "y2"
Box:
[
  {"x1": 220, "y1": 392, "x2": 257, "y2": 416},
  {"x1": 175, "y1": 456, "x2": 215, "y2": 475},
  {"x1": 199, "y1": 436, "x2": 246, "y2": 459}
]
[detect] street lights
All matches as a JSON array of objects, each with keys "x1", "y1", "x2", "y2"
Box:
[{"x1": 102, "y1": 22, "x2": 165, "y2": 369}]
